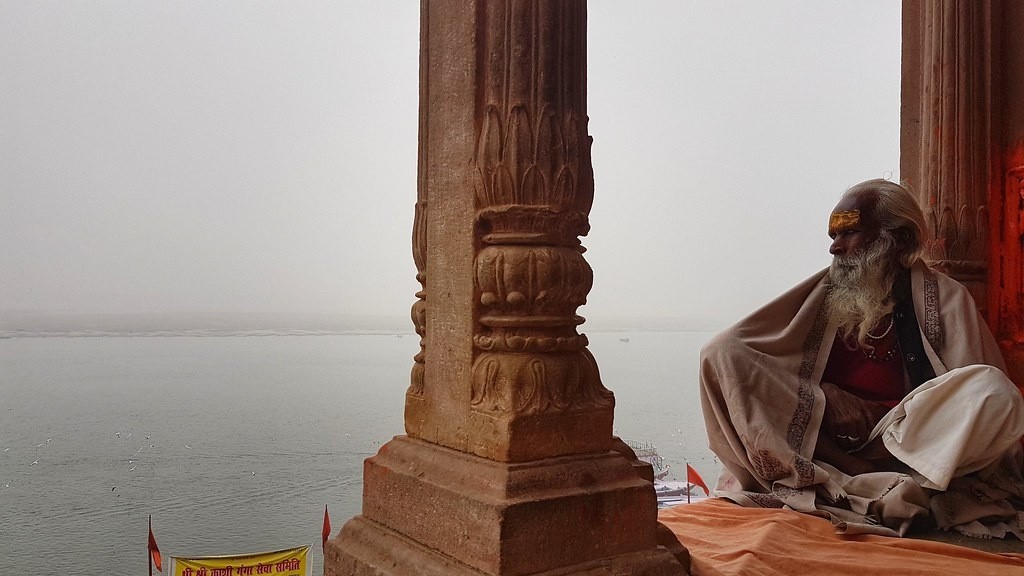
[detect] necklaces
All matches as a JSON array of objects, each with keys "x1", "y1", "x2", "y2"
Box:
[
  {"x1": 865, "y1": 342, "x2": 897, "y2": 362},
  {"x1": 867, "y1": 316, "x2": 893, "y2": 339}
]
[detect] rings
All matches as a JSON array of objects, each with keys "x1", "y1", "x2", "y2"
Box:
[
  {"x1": 848, "y1": 436, "x2": 860, "y2": 442},
  {"x1": 837, "y1": 435, "x2": 847, "y2": 438}
]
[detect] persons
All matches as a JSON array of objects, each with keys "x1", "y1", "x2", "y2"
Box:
[{"x1": 699, "y1": 178, "x2": 1024, "y2": 539}]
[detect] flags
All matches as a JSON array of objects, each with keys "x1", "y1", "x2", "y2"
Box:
[
  {"x1": 321, "y1": 512, "x2": 330, "y2": 547},
  {"x1": 147, "y1": 528, "x2": 162, "y2": 572},
  {"x1": 687, "y1": 465, "x2": 709, "y2": 497}
]
